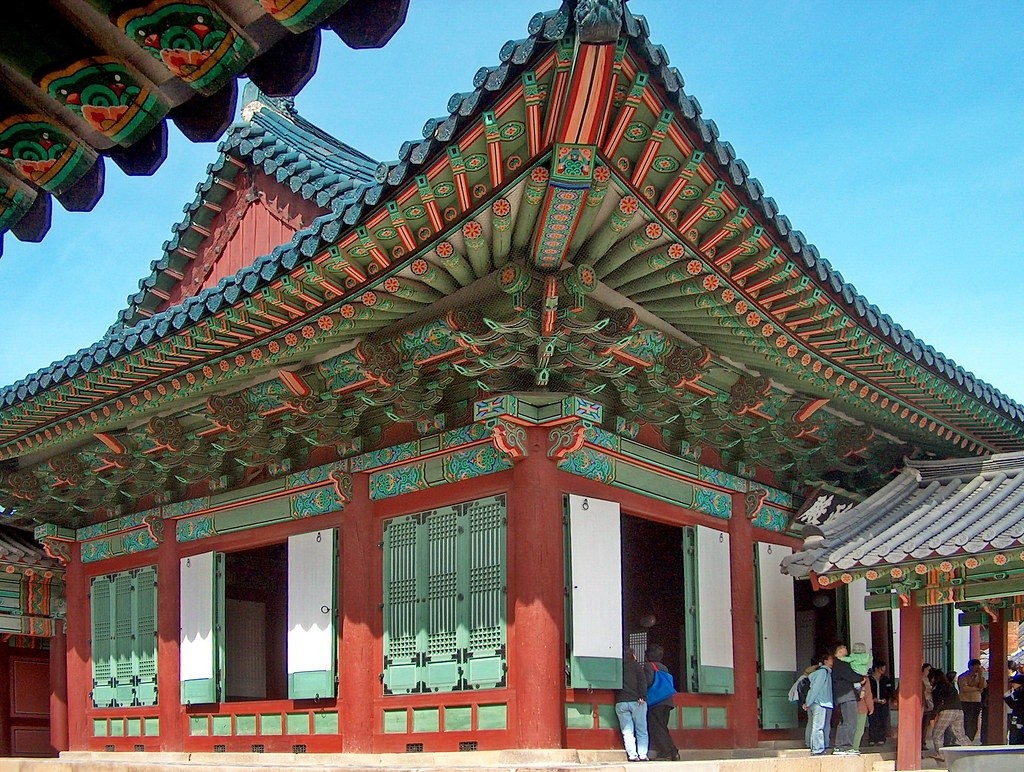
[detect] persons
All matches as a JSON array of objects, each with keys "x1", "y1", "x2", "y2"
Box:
[
  {"x1": 788, "y1": 642, "x2": 1024, "y2": 762},
  {"x1": 615, "y1": 645, "x2": 681, "y2": 762}
]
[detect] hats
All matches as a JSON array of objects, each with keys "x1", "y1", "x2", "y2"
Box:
[
  {"x1": 852, "y1": 643, "x2": 866, "y2": 653},
  {"x1": 1009, "y1": 675, "x2": 1024, "y2": 685}
]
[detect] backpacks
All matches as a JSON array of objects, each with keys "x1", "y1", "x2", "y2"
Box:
[{"x1": 797, "y1": 668, "x2": 828, "y2": 705}]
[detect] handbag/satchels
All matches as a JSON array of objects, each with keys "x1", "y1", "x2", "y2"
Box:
[{"x1": 646, "y1": 662, "x2": 677, "y2": 707}]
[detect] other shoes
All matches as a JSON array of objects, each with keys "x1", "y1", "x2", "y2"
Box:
[
  {"x1": 929, "y1": 754, "x2": 946, "y2": 762},
  {"x1": 832, "y1": 748, "x2": 862, "y2": 755},
  {"x1": 878, "y1": 741, "x2": 884, "y2": 745},
  {"x1": 630, "y1": 757, "x2": 649, "y2": 762},
  {"x1": 869, "y1": 742, "x2": 875, "y2": 745},
  {"x1": 655, "y1": 752, "x2": 680, "y2": 761},
  {"x1": 860, "y1": 690, "x2": 866, "y2": 698},
  {"x1": 811, "y1": 751, "x2": 827, "y2": 756}
]
